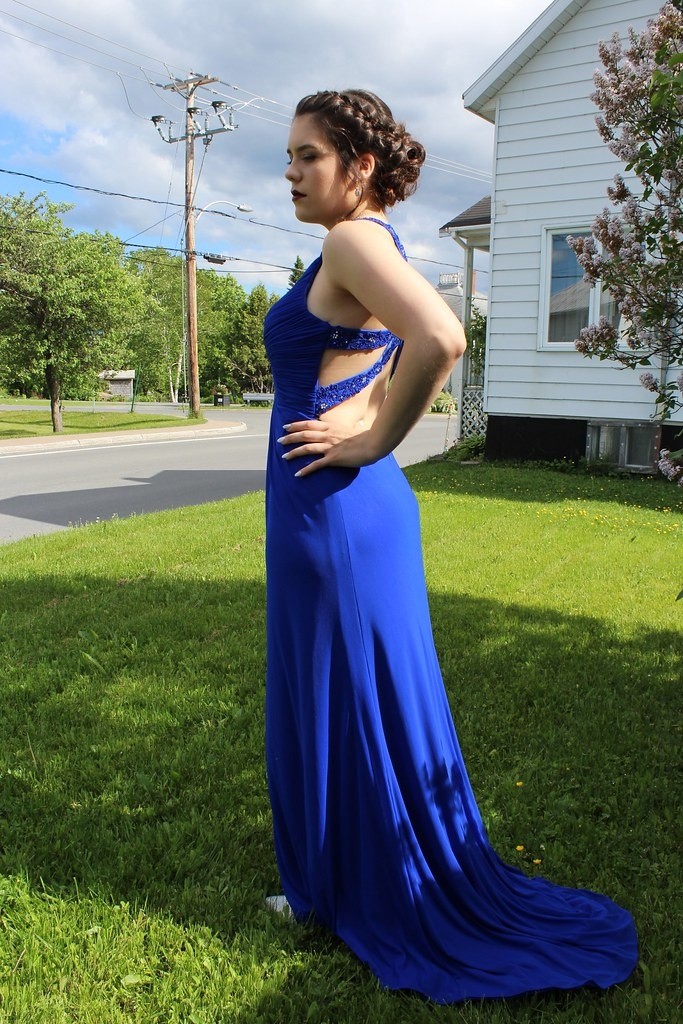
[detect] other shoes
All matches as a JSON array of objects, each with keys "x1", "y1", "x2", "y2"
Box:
[{"x1": 266, "y1": 894, "x2": 294, "y2": 919}]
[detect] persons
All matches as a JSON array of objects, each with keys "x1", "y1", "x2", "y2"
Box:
[{"x1": 264, "y1": 89, "x2": 637, "y2": 1004}]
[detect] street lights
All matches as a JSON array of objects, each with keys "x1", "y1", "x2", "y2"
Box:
[{"x1": 194, "y1": 197, "x2": 254, "y2": 229}]
